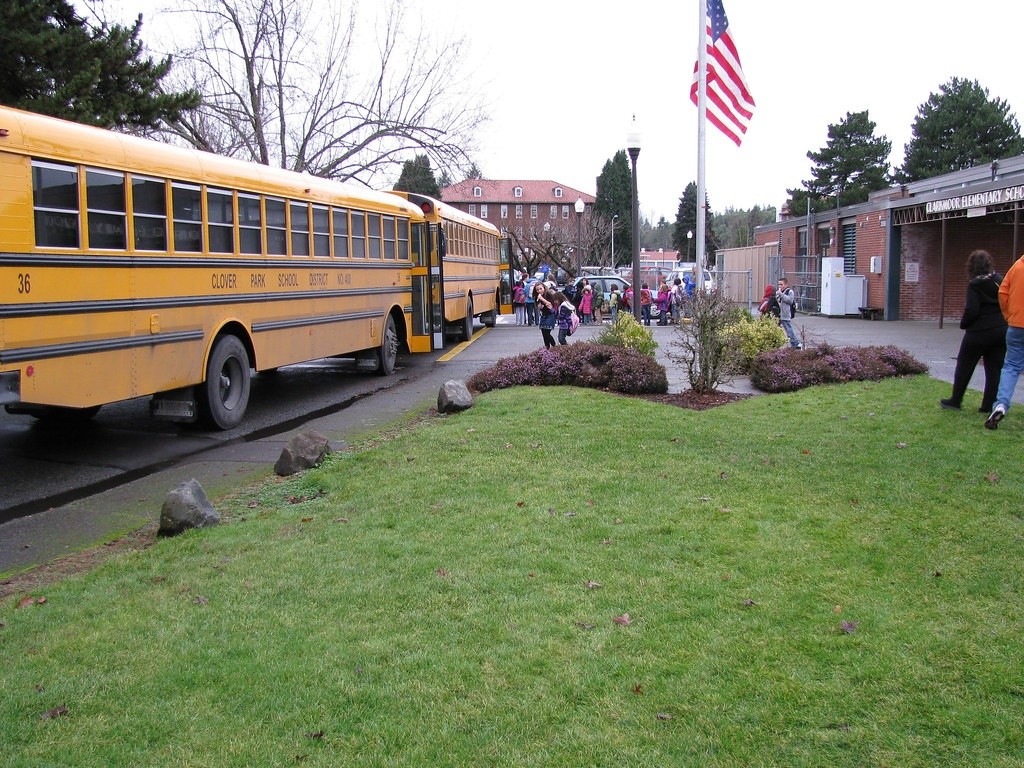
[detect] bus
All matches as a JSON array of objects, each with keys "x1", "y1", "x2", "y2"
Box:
[
  {"x1": 0, "y1": 106, "x2": 517, "y2": 439},
  {"x1": 581, "y1": 267, "x2": 632, "y2": 279}
]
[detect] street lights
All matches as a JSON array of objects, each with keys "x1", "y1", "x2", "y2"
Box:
[
  {"x1": 686, "y1": 231, "x2": 693, "y2": 264},
  {"x1": 574, "y1": 197, "x2": 585, "y2": 276},
  {"x1": 627, "y1": 114, "x2": 644, "y2": 327},
  {"x1": 611, "y1": 215, "x2": 618, "y2": 271}
]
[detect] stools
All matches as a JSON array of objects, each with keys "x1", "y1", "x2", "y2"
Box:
[{"x1": 858, "y1": 306, "x2": 880, "y2": 320}]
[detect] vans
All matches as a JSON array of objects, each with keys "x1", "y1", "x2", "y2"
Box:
[{"x1": 638, "y1": 260, "x2": 690, "y2": 269}]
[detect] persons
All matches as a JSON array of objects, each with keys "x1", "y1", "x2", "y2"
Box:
[
  {"x1": 775, "y1": 278, "x2": 801, "y2": 349},
  {"x1": 654, "y1": 274, "x2": 695, "y2": 326},
  {"x1": 940, "y1": 249, "x2": 1009, "y2": 410},
  {"x1": 985, "y1": 254, "x2": 1024, "y2": 430},
  {"x1": 756, "y1": 285, "x2": 781, "y2": 327},
  {"x1": 640, "y1": 283, "x2": 653, "y2": 326},
  {"x1": 609, "y1": 284, "x2": 633, "y2": 322},
  {"x1": 513, "y1": 273, "x2": 604, "y2": 349}
]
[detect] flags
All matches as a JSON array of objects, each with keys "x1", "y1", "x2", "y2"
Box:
[{"x1": 690, "y1": 0, "x2": 755, "y2": 148}]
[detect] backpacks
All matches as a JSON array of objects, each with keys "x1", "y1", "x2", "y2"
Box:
[
  {"x1": 514, "y1": 286, "x2": 526, "y2": 304},
  {"x1": 676, "y1": 286, "x2": 688, "y2": 302},
  {"x1": 529, "y1": 282, "x2": 537, "y2": 298},
  {"x1": 766, "y1": 295, "x2": 781, "y2": 316},
  {"x1": 785, "y1": 289, "x2": 796, "y2": 319},
  {"x1": 564, "y1": 303, "x2": 579, "y2": 336},
  {"x1": 610, "y1": 292, "x2": 622, "y2": 310},
  {"x1": 598, "y1": 297, "x2": 609, "y2": 313}
]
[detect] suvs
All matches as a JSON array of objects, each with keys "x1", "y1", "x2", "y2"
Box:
[
  {"x1": 664, "y1": 269, "x2": 715, "y2": 295},
  {"x1": 571, "y1": 276, "x2": 663, "y2": 320},
  {"x1": 624, "y1": 265, "x2": 673, "y2": 290}
]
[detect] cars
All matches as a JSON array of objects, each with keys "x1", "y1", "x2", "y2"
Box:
[{"x1": 557, "y1": 268, "x2": 571, "y2": 286}]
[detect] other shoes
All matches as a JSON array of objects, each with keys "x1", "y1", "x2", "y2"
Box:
[
  {"x1": 594, "y1": 321, "x2": 601, "y2": 325},
  {"x1": 985, "y1": 405, "x2": 1006, "y2": 429},
  {"x1": 979, "y1": 405, "x2": 992, "y2": 412},
  {"x1": 657, "y1": 322, "x2": 665, "y2": 326},
  {"x1": 940, "y1": 398, "x2": 961, "y2": 410}
]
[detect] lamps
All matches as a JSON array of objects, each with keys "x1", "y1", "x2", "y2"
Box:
[
  {"x1": 990, "y1": 160, "x2": 998, "y2": 181},
  {"x1": 900, "y1": 185, "x2": 907, "y2": 196},
  {"x1": 829, "y1": 226, "x2": 836, "y2": 236}
]
